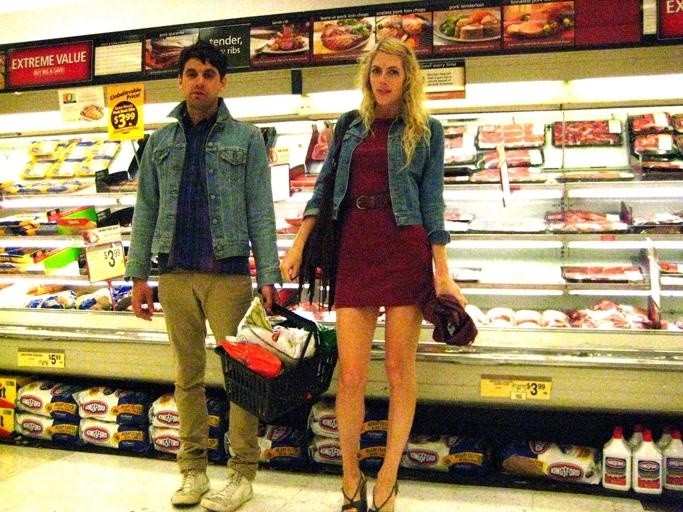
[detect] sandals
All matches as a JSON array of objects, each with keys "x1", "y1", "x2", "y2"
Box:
[{"x1": 341, "y1": 470, "x2": 399, "y2": 512}]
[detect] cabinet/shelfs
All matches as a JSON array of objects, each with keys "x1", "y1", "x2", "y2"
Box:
[{"x1": 0, "y1": 99, "x2": 682, "y2": 416}]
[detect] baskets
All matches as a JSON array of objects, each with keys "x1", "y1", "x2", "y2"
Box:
[{"x1": 214, "y1": 300, "x2": 337, "y2": 423}]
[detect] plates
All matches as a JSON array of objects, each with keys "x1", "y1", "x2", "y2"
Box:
[
  {"x1": 319, "y1": 32, "x2": 372, "y2": 52},
  {"x1": 256, "y1": 37, "x2": 310, "y2": 54},
  {"x1": 433, "y1": 27, "x2": 500, "y2": 42}
]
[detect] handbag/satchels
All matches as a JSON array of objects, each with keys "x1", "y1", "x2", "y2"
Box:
[{"x1": 297, "y1": 219, "x2": 337, "y2": 311}]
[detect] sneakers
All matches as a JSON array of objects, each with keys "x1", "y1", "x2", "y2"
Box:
[
  {"x1": 201, "y1": 469, "x2": 254, "y2": 512},
  {"x1": 171, "y1": 469, "x2": 209, "y2": 507}
]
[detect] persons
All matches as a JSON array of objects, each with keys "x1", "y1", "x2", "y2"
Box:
[
  {"x1": 123, "y1": 40, "x2": 279, "y2": 511},
  {"x1": 278, "y1": 37, "x2": 469, "y2": 512}
]
